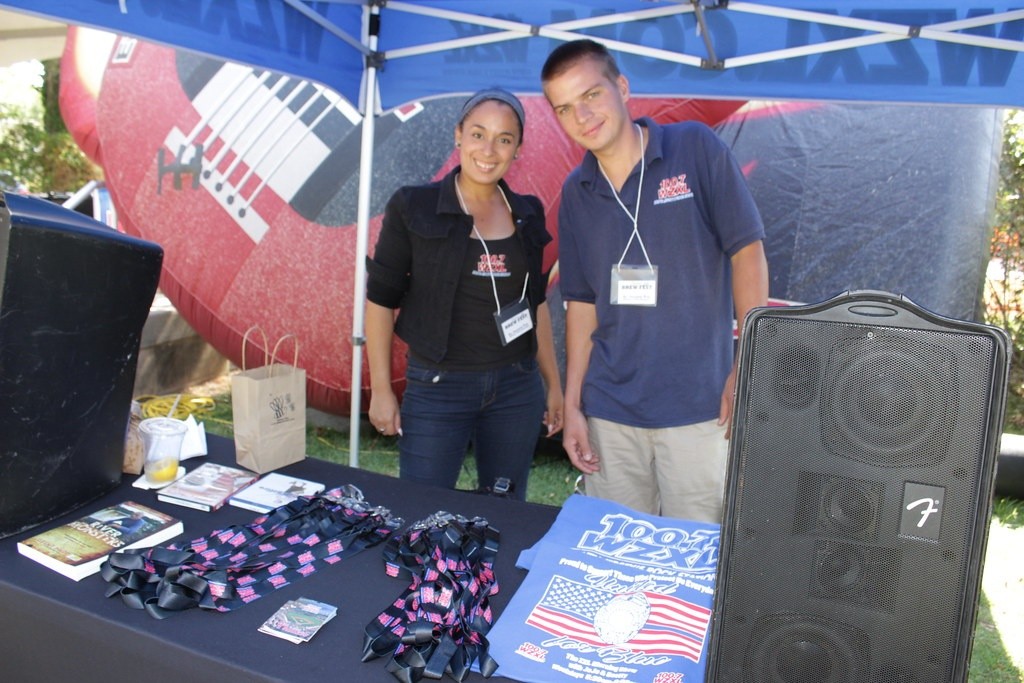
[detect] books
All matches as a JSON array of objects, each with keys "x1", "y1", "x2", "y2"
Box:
[
  {"x1": 156, "y1": 461, "x2": 260, "y2": 512},
  {"x1": 258, "y1": 596, "x2": 338, "y2": 644},
  {"x1": 226, "y1": 472, "x2": 325, "y2": 514},
  {"x1": 17, "y1": 500, "x2": 184, "y2": 583}
]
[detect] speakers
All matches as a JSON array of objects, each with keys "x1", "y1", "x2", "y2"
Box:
[
  {"x1": 0, "y1": 188, "x2": 164, "y2": 539},
  {"x1": 703, "y1": 289, "x2": 1013, "y2": 683}
]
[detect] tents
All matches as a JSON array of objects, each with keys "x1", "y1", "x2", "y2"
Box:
[{"x1": 0, "y1": 0, "x2": 1024, "y2": 468}]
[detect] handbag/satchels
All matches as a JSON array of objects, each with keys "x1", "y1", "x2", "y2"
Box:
[{"x1": 231, "y1": 325, "x2": 307, "y2": 474}]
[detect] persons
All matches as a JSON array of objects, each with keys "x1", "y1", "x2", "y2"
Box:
[
  {"x1": 365, "y1": 88, "x2": 563, "y2": 502},
  {"x1": 541, "y1": 40, "x2": 769, "y2": 525}
]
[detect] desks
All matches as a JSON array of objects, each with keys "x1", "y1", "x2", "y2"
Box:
[{"x1": 0, "y1": 433, "x2": 563, "y2": 683}]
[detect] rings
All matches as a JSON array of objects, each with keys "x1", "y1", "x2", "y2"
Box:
[{"x1": 380, "y1": 429, "x2": 385, "y2": 431}]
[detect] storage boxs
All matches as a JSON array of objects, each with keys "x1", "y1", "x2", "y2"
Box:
[{"x1": 231, "y1": 363, "x2": 306, "y2": 474}]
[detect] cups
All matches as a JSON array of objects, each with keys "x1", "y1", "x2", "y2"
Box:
[{"x1": 138, "y1": 417, "x2": 188, "y2": 482}]
